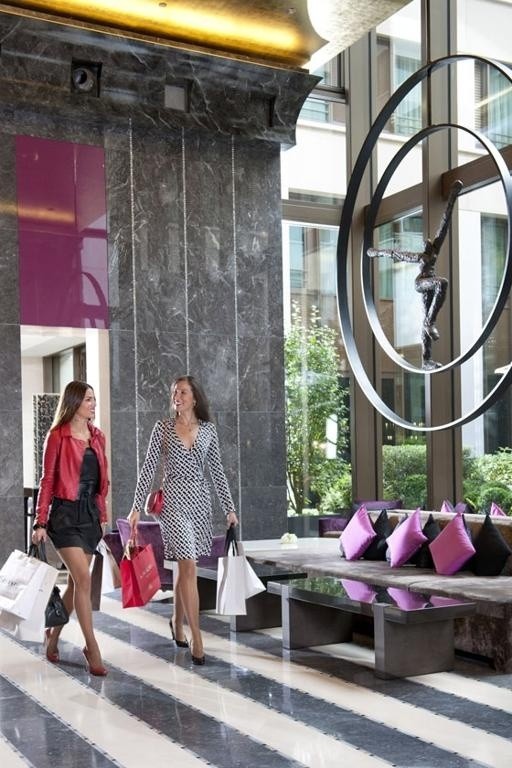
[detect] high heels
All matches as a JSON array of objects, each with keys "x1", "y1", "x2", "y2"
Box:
[
  {"x1": 43, "y1": 628, "x2": 60, "y2": 663},
  {"x1": 190, "y1": 639, "x2": 205, "y2": 665},
  {"x1": 169, "y1": 618, "x2": 190, "y2": 648},
  {"x1": 82, "y1": 646, "x2": 108, "y2": 676}
]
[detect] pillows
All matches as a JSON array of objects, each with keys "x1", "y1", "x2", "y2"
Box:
[{"x1": 337, "y1": 504, "x2": 510, "y2": 579}]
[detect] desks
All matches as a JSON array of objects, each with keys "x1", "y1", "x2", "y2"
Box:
[
  {"x1": 163, "y1": 557, "x2": 310, "y2": 633},
  {"x1": 268, "y1": 576, "x2": 479, "y2": 682}
]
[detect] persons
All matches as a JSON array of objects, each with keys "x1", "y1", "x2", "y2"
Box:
[
  {"x1": 125, "y1": 375, "x2": 239, "y2": 665},
  {"x1": 383, "y1": 205, "x2": 446, "y2": 370},
  {"x1": 28, "y1": 379, "x2": 108, "y2": 678}
]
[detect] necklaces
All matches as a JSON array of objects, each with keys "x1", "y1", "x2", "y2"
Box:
[{"x1": 180, "y1": 418, "x2": 193, "y2": 433}]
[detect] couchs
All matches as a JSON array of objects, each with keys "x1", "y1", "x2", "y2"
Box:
[{"x1": 232, "y1": 515, "x2": 511, "y2": 673}]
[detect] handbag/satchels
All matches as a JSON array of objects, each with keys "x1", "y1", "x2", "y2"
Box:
[
  {"x1": 88, "y1": 538, "x2": 104, "y2": 613},
  {"x1": 98, "y1": 538, "x2": 122, "y2": 595},
  {"x1": 34, "y1": 538, "x2": 70, "y2": 628},
  {"x1": 119, "y1": 523, "x2": 162, "y2": 609},
  {"x1": 144, "y1": 489, "x2": 163, "y2": 517},
  {"x1": 227, "y1": 523, "x2": 267, "y2": 600},
  {"x1": 215, "y1": 523, "x2": 248, "y2": 617},
  {"x1": 0, "y1": 537, "x2": 47, "y2": 620},
  {"x1": 0, "y1": 537, "x2": 60, "y2": 644}
]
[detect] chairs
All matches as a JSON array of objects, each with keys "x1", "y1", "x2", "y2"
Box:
[{"x1": 102, "y1": 520, "x2": 224, "y2": 587}]
